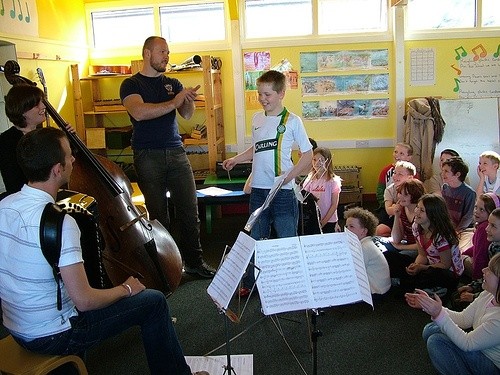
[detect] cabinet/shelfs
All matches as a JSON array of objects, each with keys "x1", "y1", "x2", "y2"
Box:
[{"x1": 71, "y1": 55, "x2": 226, "y2": 180}]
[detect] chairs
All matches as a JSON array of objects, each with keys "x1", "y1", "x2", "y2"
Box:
[{"x1": 0, "y1": 333, "x2": 89, "y2": 375}]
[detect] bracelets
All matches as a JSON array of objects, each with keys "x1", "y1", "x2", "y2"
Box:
[{"x1": 120, "y1": 283, "x2": 132, "y2": 297}]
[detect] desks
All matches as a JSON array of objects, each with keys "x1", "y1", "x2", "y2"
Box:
[{"x1": 204, "y1": 173, "x2": 248, "y2": 236}]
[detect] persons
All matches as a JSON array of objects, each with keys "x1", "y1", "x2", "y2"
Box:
[
  {"x1": 1, "y1": 126, "x2": 209, "y2": 375},
  {"x1": 120, "y1": 37, "x2": 217, "y2": 278},
  {"x1": 243, "y1": 139, "x2": 342, "y2": 234},
  {"x1": 405, "y1": 252, "x2": 500, "y2": 375},
  {"x1": 343, "y1": 206, "x2": 392, "y2": 303},
  {"x1": 221, "y1": 71, "x2": 314, "y2": 297},
  {"x1": 0, "y1": 82, "x2": 114, "y2": 290},
  {"x1": 372, "y1": 143, "x2": 500, "y2": 333}
]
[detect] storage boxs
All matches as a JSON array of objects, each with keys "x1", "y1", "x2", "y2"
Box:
[
  {"x1": 87, "y1": 126, "x2": 133, "y2": 152},
  {"x1": 332, "y1": 166, "x2": 378, "y2": 209}
]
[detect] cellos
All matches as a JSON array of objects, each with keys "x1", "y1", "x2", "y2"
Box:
[{"x1": 5, "y1": 59, "x2": 182, "y2": 300}]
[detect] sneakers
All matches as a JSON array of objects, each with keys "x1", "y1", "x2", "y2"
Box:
[{"x1": 184, "y1": 259, "x2": 217, "y2": 277}]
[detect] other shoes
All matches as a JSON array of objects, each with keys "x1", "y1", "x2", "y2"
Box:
[
  {"x1": 391, "y1": 278, "x2": 400, "y2": 287},
  {"x1": 236, "y1": 288, "x2": 256, "y2": 297},
  {"x1": 423, "y1": 287, "x2": 447, "y2": 299}
]
